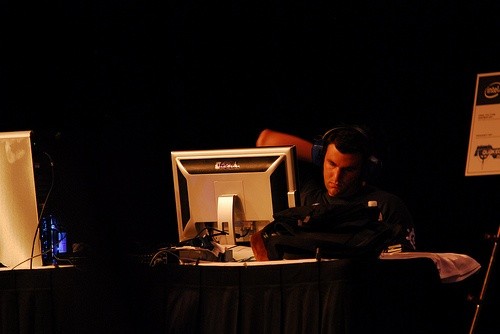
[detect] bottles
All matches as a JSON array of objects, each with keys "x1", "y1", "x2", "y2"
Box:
[{"x1": 41, "y1": 215, "x2": 72, "y2": 265}]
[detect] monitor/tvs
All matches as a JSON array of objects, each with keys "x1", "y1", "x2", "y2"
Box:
[{"x1": 172, "y1": 145, "x2": 300, "y2": 247}]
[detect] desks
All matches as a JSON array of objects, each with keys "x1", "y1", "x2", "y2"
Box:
[
  {"x1": 151, "y1": 249, "x2": 487, "y2": 334},
  {"x1": 0, "y1": 265, "x2": 74, "y2": 334}
]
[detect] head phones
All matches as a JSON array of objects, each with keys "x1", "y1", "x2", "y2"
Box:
[{"x1": 313, "y1": 126, "x2": 379, "y2": 171}]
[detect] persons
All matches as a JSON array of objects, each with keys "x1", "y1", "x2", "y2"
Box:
[{"x1": 256, "y1": 121, "x2": 416, "y2": 252}]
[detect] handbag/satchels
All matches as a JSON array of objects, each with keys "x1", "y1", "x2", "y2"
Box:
[{"x1": 262, "y1": 200, "x2": 401, "y2": 259}]
